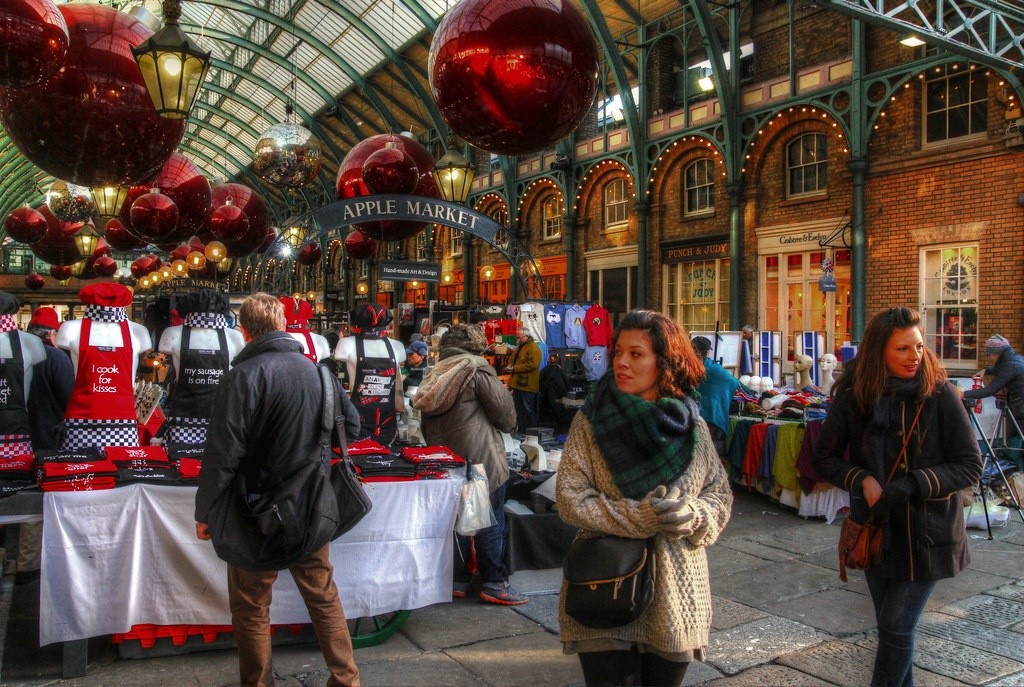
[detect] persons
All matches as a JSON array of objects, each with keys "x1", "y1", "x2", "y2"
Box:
[
  {"x1": 554, "y1": 311, "x2": 734, "y2": 687},
  {"x1": 504, "y1": 327, "x2": 542, "y2": 433},
  {"x1": 279, "y1": 296, "x2": 331, "y2": 363},
  {"x1": 0, "y1": 291, "x2": 47, "y2": 459},
  {"x1": 689, "y1": 335, "x2": 739, "y2": 442},
  {"x1": 958, "y1": 333, "x2": 1024, "y2": 460},
  {"x1": 402, "y1": 340, "x2": 429, "y2": 393},
  {"x1": 739, "y1": 375, "x2": 773, "y2": 392},
  {"x1": 742, "y1": 325, "x2": 754, "y2": 355},
  {"x1": 158, "y1": 289, "x2": 245, "y2": 457},
  {"x1": 26, "y1": 307, "x2": 75, "y2": 415},
  {"x1": 411, "y1": 324, "x2": 529, "y2": 604},
  {"x1": 193, "y1": 291, "x2": 361, "y2": 687},
  {"x1": 820, "y1": 353, "x2": 837, "y2": 395},
  {"x1": 795, "y1": 354, "x2": 814, "y2": 392},
  {"x1": 335, "y1": 302, "x2": 406, "y2": 444},
  {"x1": 57, "y1": 282, "x2": 152, "y2": 451},
  {"x1": 324, "y1": 324, "x2": 340, "y2": 349},
  {"x1": 813, "y1": 306, "x2": 983, "y2": 687}
]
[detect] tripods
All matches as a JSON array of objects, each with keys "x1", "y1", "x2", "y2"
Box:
[{"x1": 958, "y1": 397, "x2": 1024, "y2": 541}]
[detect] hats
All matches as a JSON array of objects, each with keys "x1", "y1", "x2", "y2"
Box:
[
  {"x1": 691, "y1": 337, "x2": 712, "y2": 352},
  {"x1": 439, "y1": 323, "x2": 488, "y2": 354},
  {"x1": 28, "y1": 307, "x2": 60, "y2": 330},
  {"x1": 175, "y1": 288, "x2": 229, "y2": 329},
  {"x1": 78, "y1": 281, "x2": 133, "y2": 322},
  {"x1": 350, "y1": 303, "x2": 394, "y2": 339},
  {"x1": 405, "y1": 340, "x2": 428, "y2": 356},
  {"x1": 985, "y1": 334, "x2": 1010, "y2": 354},
  {"x1": 0, "y1": 291, "x2": 19, "y2": 332},
  {"x1": 410, "y1": 333, "x2": 422, "y2": 342},
  {"x1": 278, "y1": 297, "x2": 314, "y2": 333}
]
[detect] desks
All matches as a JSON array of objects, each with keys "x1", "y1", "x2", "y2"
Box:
[
  {"x1": 40, "y1": 473, "x2": 464, "y2": 677},
  {"x1": 725, "y1": 415, "x2": 850, "y2": 522}
]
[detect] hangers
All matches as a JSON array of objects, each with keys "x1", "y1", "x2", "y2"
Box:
[{"x1": 526, "y1": 298, "x2": 599, "y2": 308}]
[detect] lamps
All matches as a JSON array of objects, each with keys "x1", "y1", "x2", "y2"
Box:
[
  {"x1": 291, "y1": 257, "x2": 544, "y2": 302},
  {"x1": 59, "y1": 280, "x2": 68, "y2": 286},
  {"x1": 89, "y1": 185, "x2": 130, "y2": 217},
  {"x1": 139, "y1": 240, "x2": 233, "y2": 289},
  {"x1": 431, "y1": 125, "x2": 476, "y2": 204},
  {"x1": 897, "y1": 30, "x2": 927, "y2": 48},
  {"x1": 280, "y1": 0, "x2": 311, "y2": 249},
  {"x1": 128, "y1": 0, "x2": 214, "y2": 118},
  {"x1": 72, "y1": 215, "x2": 101, "y2": 259},
  {"x1": 697, "y1": 76, "x2": 716, "y2": 91},
  {"x1": 611, "y1": 108, "x2": 625, "y2": 122},
  {"x1": 70, "y1": 260, "x2": 86, "y2": 276}
]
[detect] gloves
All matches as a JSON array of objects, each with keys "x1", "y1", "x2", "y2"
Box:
[
  {"x1": 884, "y1": 477, "x2": 918, "y2": 503},
  {"x1": 648, "y1": 485, "x2": 697, "y2": 540}
]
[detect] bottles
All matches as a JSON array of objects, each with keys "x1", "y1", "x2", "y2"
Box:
[
  {"x1": 343, "y1": 382, "x2": 348, "y2": 390},
  {"x1": 973, "y1": 377, "x2": 982, "y2": 389}
]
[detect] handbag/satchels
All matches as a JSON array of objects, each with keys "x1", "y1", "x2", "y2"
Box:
[
  {"x1": 330, "y1": 458, "x2": 372, "y2": 542},
  {"x1": 228, "y1": 459, "x2": 339, "y2": 565},
  {"x1": 839, "y1": 518, "x2": 884, "y2": 582},
  {"x1": 563, "y1": 534, "x2": 657, "y2": 628}
]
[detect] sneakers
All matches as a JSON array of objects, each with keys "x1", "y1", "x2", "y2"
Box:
[
  {"x1": 479, "y1": 586, "x2": 529, "y2": 605},
  {"x1": 453, "y1": 580, "x2": 471, "y2": 598}
]
